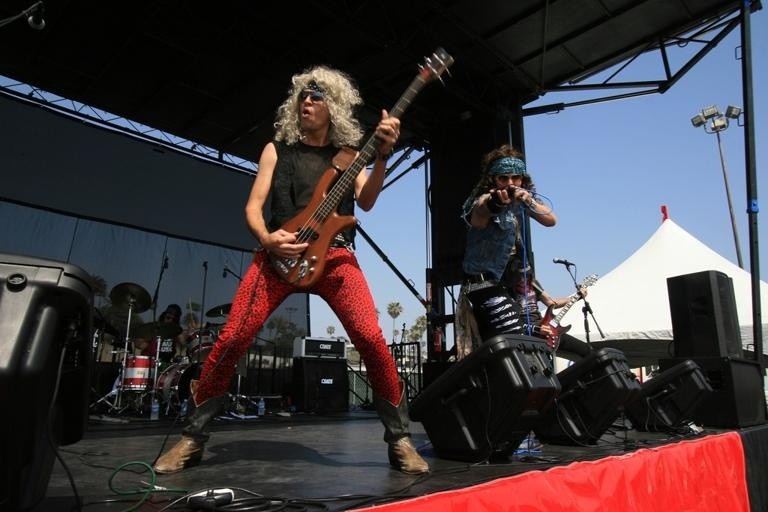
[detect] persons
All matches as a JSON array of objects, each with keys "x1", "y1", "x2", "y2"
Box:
[
  {"x1": 514, "y1": 266, "x2": 587, "y2": 337},
  {"x1": 136, "y1": 303, "x2": 187, "y2": 354},
  {"x1": 153, "y1": 67, "x2": 431, "y2": 472},
  {"x1": 463, "y1": 147, "x2": 556, "y2": 336}
]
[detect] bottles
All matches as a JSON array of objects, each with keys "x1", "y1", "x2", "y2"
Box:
[
  {"x1": 258, "y1": 397, "x2": 266, "y2": 416},
  {"x1": 151, "y1": 399, "x2": 161, "y2": 421},
  {"x1": 180, "y1": 398, "x2": 187, "y2": 422}
]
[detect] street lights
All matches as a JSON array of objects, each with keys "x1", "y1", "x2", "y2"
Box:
[{"x1": 692, "y1": 101, "x2": 746, "y2": 269}]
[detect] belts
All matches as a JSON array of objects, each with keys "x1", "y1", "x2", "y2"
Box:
[{"x1": 330, "y1": 231, "x2": 348, "y2": 248}]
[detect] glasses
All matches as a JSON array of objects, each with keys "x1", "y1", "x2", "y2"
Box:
[
  {"x1": 498, "y1": 173, "x2": 523, "y2": 184},
  {"x1": 166, "y1": 315, "x2": 180, "y2": 321},
  {"x1": 296, "y1": 89, "x2": 328, "y2": 106}
]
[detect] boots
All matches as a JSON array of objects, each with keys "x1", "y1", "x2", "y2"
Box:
[
  {"x1": 371, "y1": 378, "x2": 431, "y2": 476},
  {"x1": 150, "y1": 377, "x2": 230, "y2": 476}
]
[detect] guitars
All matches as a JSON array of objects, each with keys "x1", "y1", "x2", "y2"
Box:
[
  {"x1": 266, "y1": 46, "x2": 455, "y2": 291},
  {"x1": 537, "y1": 273, "x2": 598, "y2": 352}
]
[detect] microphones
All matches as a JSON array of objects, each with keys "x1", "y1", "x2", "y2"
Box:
[
  {"x1": 505, "y1": 186, "x2": 530, "y2": 207},
  {"x1": 165, "y1": 252, "x2": 168, "y2": 268},
  {"x1": 553, "y1": 258, "x2": 575, "y2": 266},
  {"x1": 223, "y1": 262, "x2": 228, "y2": 277},
  {"x1": 27, "y1": 3, "x2": 45, "y2": 30}
]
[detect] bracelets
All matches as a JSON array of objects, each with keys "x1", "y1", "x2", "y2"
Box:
[{"x1": 530, "y1": 199, "x2": 537, "y2": 209}]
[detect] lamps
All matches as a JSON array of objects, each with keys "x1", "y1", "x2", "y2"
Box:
[{"x1": 688, "y1": 104, "x2": 741, "y2": 132}]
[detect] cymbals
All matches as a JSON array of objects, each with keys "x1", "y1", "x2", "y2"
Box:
[
  {"x1": 207, "y1": 302, "x2": 233, "y2": 317},
  {"x1": 109, "y1": 282, "x2": 151, "y2": 314}
]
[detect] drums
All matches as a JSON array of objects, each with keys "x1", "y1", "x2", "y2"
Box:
[
  {"x1": 120, "y1": 355, "x2": 156, "y2": 391},
  {"x1": 187, "y1": 328, "x2": 218, "y2": 362},
  {"x1": 156, "y1": 361, "x2": 204, "y2": 408}
]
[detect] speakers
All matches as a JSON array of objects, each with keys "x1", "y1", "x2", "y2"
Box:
[
  {"x1": 408, "y1": 334, "x2": 563, "y2": 466},
  {"x1": 663, "y1": 357, "x2": 768, "y2": 427},
  {"x1": 532, "y1": 347, "x2": 642, "y2": 446},
  {"x1": 0, "y1": 252, "x2": 93, "y2": 512},
  {"x1": 292, "y1": 357, "x2": 350, "y2": 412},
  {"x1": 626, "y1": 361, "x2": 714, "y2": 431},
  {"x1": 667, "y1": 270, "x2": 744, "y2": 358}
]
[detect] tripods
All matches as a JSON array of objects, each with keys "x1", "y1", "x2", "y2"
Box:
[
  {"x1": 212, "y1": 336, "x2": 275, "y2": 421},
  {"x1": 90, "y1": 303, "x2": 180, "y2": 419}
]
